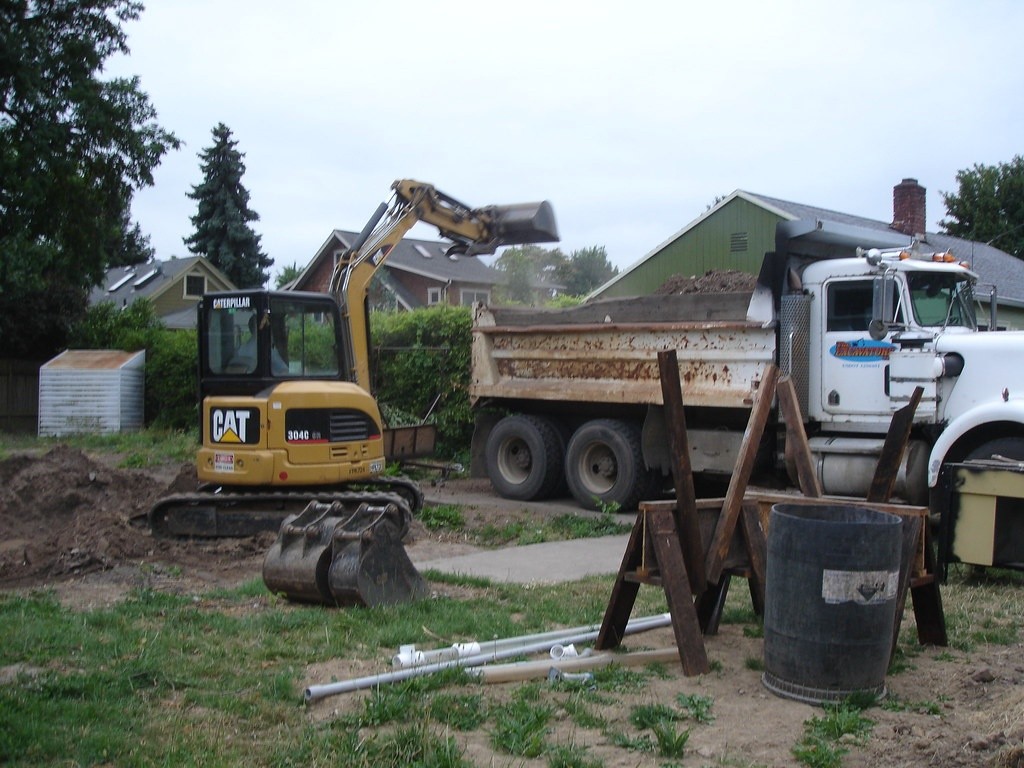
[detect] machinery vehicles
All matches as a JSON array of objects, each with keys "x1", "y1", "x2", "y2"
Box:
[{"x1": 145, "y1": 178, "x2": 562, "y2": 537}]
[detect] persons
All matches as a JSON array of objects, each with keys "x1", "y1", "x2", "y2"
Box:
[{"x1": 239, "y1": 314, "x2": 290, "y2": 374}]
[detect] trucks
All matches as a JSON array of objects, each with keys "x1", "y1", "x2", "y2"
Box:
[{"x1": 466, "y1": 239, "x2": 1023, "y2": 568}]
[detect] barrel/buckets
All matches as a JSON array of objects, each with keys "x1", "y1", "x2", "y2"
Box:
[{"x1": 759, "y1": 501, "x2": 903, "y2": 708}]
